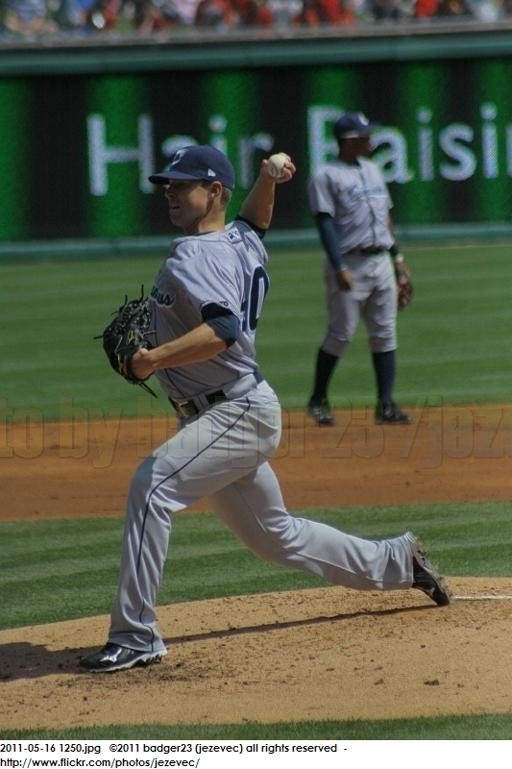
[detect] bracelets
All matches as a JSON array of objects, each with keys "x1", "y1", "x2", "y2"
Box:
[
  {"x1": 334, "y1": 265, "x2": 349, "y2": 275},
  {"x1": 394, "y1": 254, "x2": 405, "y2": 265}
]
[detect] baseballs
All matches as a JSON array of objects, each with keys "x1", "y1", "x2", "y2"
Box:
[{"x1": 266, "y1": 152, "x2": 292, "y2": 179}]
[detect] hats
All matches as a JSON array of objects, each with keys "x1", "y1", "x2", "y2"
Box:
[
  {"x1": 331, "y1": 112, "x2": 379, "y2": 139},
  {"x1": 147, "y1": 144, "x2": 233, "y2": 191}
]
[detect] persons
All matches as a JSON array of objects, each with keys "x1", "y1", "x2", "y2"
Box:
[
  {"x1": 72, "y1": 141, "x2": 455, "y2": 677},
  {"x1": 302, "y1": 109, "x2": 418, "y2": 430}
]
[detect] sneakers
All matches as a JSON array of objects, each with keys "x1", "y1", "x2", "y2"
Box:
[
  {"x1": 307, "y1": 397, "x2": 335, "y2": 427},
  {"x1": 404, "y1": 527, "x2": 454, "y2": 606},
  {"x1": 84, "y1": 642, "x2": 168, "y2": 673},
  {"x1": 374, "y1": 402, "x2": 411, "y2": 423}
]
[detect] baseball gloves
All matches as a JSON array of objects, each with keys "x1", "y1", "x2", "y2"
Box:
[
  {"x1": 102, "y1": 298, "x2": 155, "y2": 384},
  {"x1": 394, "y1": 261, "x2": 415, "y2": 310}
]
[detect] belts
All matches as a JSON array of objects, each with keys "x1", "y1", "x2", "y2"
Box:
[
  {"x1": 351, "y1": 245, "x2": 385, "y2": 255},
  {"x1": 168, "y1": 370, "x2": 264, "y2": 418}
]
